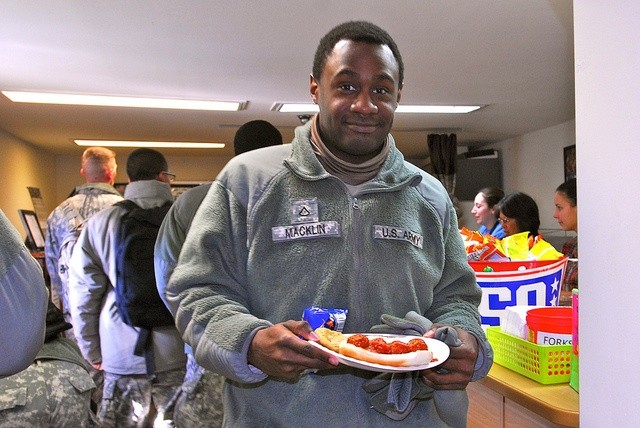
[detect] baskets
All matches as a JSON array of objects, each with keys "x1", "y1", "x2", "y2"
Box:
[{"x1": 486, "y1": 326, "x2": 572, "y2": 385}]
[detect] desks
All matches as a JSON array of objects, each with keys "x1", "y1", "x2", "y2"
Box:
[{"x1": 466, "y1": 362, "x2": 577, "y2": 427}]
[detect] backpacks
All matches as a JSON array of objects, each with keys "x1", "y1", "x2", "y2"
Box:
[
  {"x1": 57, "y1": 198, "x2": 89, "y2": 323},
  {"x1": 111, "y1": 200, "x2": 176, "y2": 375}
]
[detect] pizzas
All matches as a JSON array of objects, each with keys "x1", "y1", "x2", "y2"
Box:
[{"x1": 314, "y1": 327, "x2": 433, "y2": 368}]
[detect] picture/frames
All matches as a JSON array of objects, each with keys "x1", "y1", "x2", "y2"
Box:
[
  {"x1": 563, "y1": 144, "x2": 576, "y2": 183},
  {"x1": 19, "y1": 210, "x2": 45, "y2": 249}
]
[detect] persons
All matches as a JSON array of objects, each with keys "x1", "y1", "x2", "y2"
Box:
[
  {"x1": 153, "y1": 120, "x2": 283, "y2": 426},
  {"x1": 165, "y1": 20, "x2": 493, "y2": 427},
  {"x1": 495, "y1": 193, "x2": 542, "y2": 235},
  {"x1": 68, "y1": 148, "x2": 185, "y2": 428},
  {"x1": 1, "y1": 303, "x2": 96, "y2": 428},
  {"x1": 2, "y1": 210, "x2": 51, "y2": 377},
  {"x1": 472, "y1": 187, "x2": 505, "y2": 237},
  {"x1": 45, "y1": 147, "x2": 125, "y2": 319},
  {"x1": 551, "y1": 179, "x2": 577, "y2": 299}
]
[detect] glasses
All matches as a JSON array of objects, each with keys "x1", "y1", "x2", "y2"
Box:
[
  {"x1": 498, "y1": 217, "x2": 514, "y2": 223},
  {"x1": 163, "y1": 172, "x2": 176, "y2": 183}
]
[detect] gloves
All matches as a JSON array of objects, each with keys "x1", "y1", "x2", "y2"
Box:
[{"x1": 363, "y1": 311, "x2": 462, "y2": 421}]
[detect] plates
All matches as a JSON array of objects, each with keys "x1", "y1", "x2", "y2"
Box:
[{"x1": 307, "y1": 333, "x2": 450, "y2": 374}]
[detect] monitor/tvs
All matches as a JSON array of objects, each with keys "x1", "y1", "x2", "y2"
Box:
[{"x1": 18, "y1": 210, "x2": 46, "y2": 252}]
